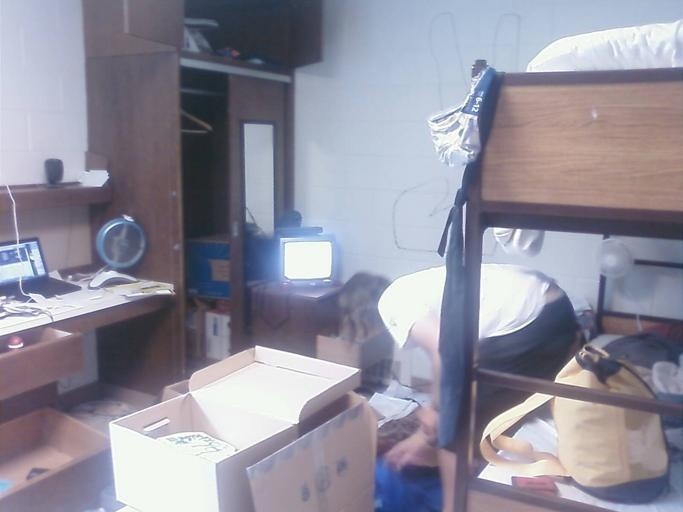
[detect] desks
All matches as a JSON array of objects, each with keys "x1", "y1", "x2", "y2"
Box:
[
  {"x1": 248, "y1": 280, "x2": 337, "y2": 352},
  {"x1": 0, "y1": 268, "x2": 175, "y2": 430}
]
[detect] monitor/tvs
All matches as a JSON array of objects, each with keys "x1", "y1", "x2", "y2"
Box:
[{"x1": 279, "y1": 235, "x2": 340, "y2": 288}]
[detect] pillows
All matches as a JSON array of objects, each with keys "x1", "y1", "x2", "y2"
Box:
[{"x1": 519, "y1": 19, "x2": 681, "y2": 72}]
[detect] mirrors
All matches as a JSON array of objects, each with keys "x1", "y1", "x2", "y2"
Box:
[{"x1": 238, "y1": 118, "x2": 279, "y2": 332}]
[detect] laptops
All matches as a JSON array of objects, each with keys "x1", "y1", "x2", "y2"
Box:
[{"x1": 0, "y1": 237, "x2": 82, "y2": 305}]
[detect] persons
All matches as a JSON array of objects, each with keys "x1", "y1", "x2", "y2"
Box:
[{"x1": 328, "y1": 261, "x2": 581, "y2": 511}]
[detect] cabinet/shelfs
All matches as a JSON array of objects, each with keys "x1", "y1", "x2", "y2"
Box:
[{"x1": 81, "y1": 2, "x2": 325, "y2": 406}]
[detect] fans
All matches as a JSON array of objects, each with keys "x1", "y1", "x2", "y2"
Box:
[
  {"x1": 84, "y1": 212, "x2": 145, "y2": 286},
  {"x1": 590, "y1": 236, "x2": 636, "y2": 282}
]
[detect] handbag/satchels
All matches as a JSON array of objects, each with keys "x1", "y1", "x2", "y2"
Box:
[{"x1": 480, "y1": 341, "x2": 672, "y2": 507}]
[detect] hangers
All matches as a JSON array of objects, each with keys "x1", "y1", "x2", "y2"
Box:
[{"x1": 178, "y1": 101, "x2": 215, "y2": 135}]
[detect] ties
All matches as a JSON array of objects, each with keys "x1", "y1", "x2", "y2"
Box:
[{"x1": 428, "y1": 72, "x2": 503, "y2": 450}]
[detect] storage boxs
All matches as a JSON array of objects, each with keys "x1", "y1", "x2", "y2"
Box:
[
  {"x1": 0, "y1": 327, "x2": 401, "y2": 511},
  {"x1": 185, "y1": 232, "x2": 235, "y2": 300}
]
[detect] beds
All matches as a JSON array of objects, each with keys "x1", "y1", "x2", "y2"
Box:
[{"x1": 435, "y1": 14, "x2": 683, "y2": 510}]
